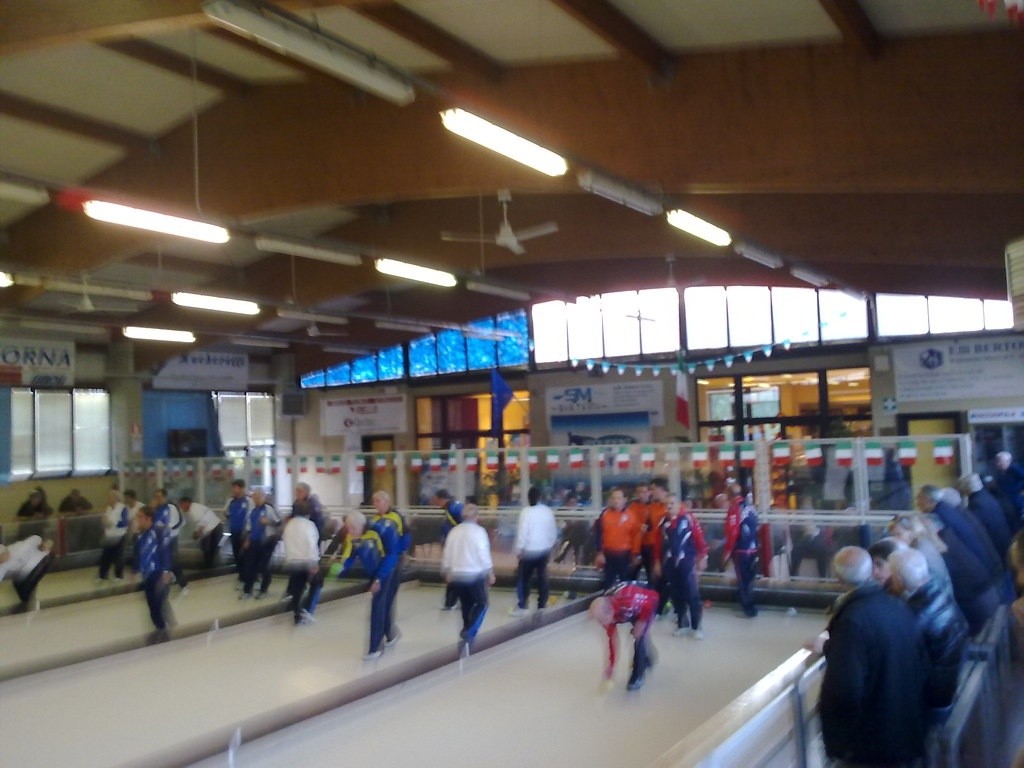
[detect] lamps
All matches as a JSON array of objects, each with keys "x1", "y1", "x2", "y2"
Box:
[{"x1": 0, "y1": 0, "x2": 868, "y2": 355}]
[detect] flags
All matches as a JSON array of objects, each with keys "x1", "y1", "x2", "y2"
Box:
[
  {"x1": 486, "y1": 446, "x2": 679, "y2": 471},
  {"x1": 356, "y1": 451, "x2": 478, "y2": 471},
  {"x1": 676, "y1": 351, "x2": 689, "y2": 430},
  {"x1": 491, "y1": 369, "x2": 513, "y2": 438},
  {"x1": 124, "y1": 455, "x2": 340, "y2": 478},
  {"x1": 692, "y1": 438, "x2": 954, "y2": 468}
]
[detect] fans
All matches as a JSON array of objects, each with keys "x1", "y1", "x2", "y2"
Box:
[
  {"x1": 282, "y1": 323, "x2": 348, "y2": 339},
  {"x1": 39, "y1": 273, "x2": 139, "y2": 316},
  {"x1": 440, "y1": 187, "x2": 558, "y2": 256}
]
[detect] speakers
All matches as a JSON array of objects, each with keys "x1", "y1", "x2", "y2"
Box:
[{"x1": 168, "y1": 428, "x2": 209, "y2": 459}]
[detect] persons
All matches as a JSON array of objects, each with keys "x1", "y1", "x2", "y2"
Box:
[
  {"x1": 720, "y1": 484, "x2": 761, "y2": 617},
  {"x1": 597, "y1": 478, "x2": 708, "y2": 640},
  {"x1": 805, "y1": 453, "x2": 1024, "y2": 768},
  {"x1": 0, "y1": 535, "x2": 54, "y2": 612},
  {"x1": 17, "y1": 491, "x2": 54, "y2": 538},
  {"x1": 441, "y1": 504, "x2": 497, "y2": 657},
  {"x1": 59, "y1": 489, "x2": 93, "y2": 515},
  {"x1": 96, "y1": 488, "x2": 223, "y2": 642},
  {"x1": 511, "y1": 487, "x2": 557, "y2": 614},
  {"x1": 222, "y1": 480, "x2": 411, "y2": 661},
  {"x1": 590, "y1": 580, "x2": 659, "y2": 690},
  {"x1": 435, "y1": 488, "x2": 465, "y2": 611},
  {"x1": 545, "y1": 481, "x2": 591, "y2": 564}
]
